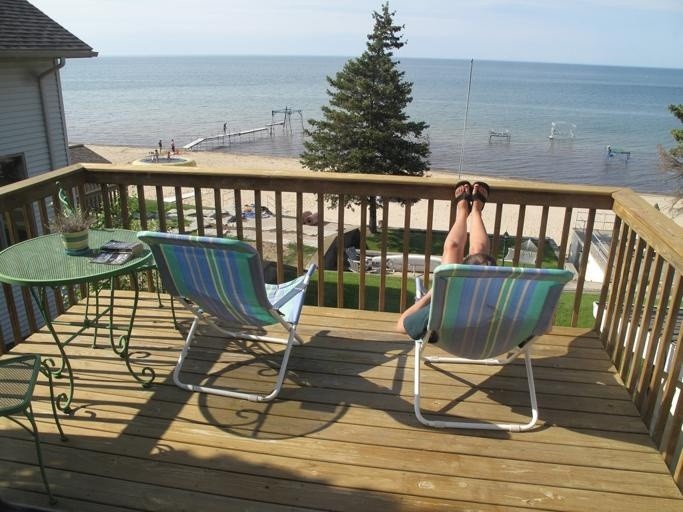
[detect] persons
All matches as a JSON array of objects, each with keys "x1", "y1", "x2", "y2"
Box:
[
  {"x1": 223, "y1": 122, "x2": 227, "y2": 135},
  {"x1": 396, "y1": 180, "x2": 498, "y2": 341},
  {"x1": 154, "y1": 138, "x2": 175, "y2": 160}
]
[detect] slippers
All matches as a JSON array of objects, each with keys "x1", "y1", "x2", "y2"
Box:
[{"x1": 453, "y1": 180, "x2": 490, "y2": 212}]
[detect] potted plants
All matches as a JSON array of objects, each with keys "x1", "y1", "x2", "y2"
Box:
[{"x1": 43, "y1": 181, "x2": 91, "y2": 256}]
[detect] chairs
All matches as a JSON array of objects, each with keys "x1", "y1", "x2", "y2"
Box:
[
  {"x1": 413, "y1": 264, "x2": 575, "y2": 430},
  {"x1": 136, "y1": 231, "x2": 317, "y2": 404}
]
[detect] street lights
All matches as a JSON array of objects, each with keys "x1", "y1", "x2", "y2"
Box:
[{"x1": 501, "y1": 229, "x2": 508, "y2": 265}]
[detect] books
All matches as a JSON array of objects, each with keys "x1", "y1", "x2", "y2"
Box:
[{"x1": 89, "y1": 240, "x2": 144, "y2": 265}]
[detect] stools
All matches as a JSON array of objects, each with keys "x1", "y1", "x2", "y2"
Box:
[{"x1": 0, "y1": 353, "x2": 69, "y2": 505}]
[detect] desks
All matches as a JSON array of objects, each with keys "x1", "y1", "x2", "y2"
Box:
[{"x1": 0, "y1": 229, "x2": 156, "y2": 413}]
